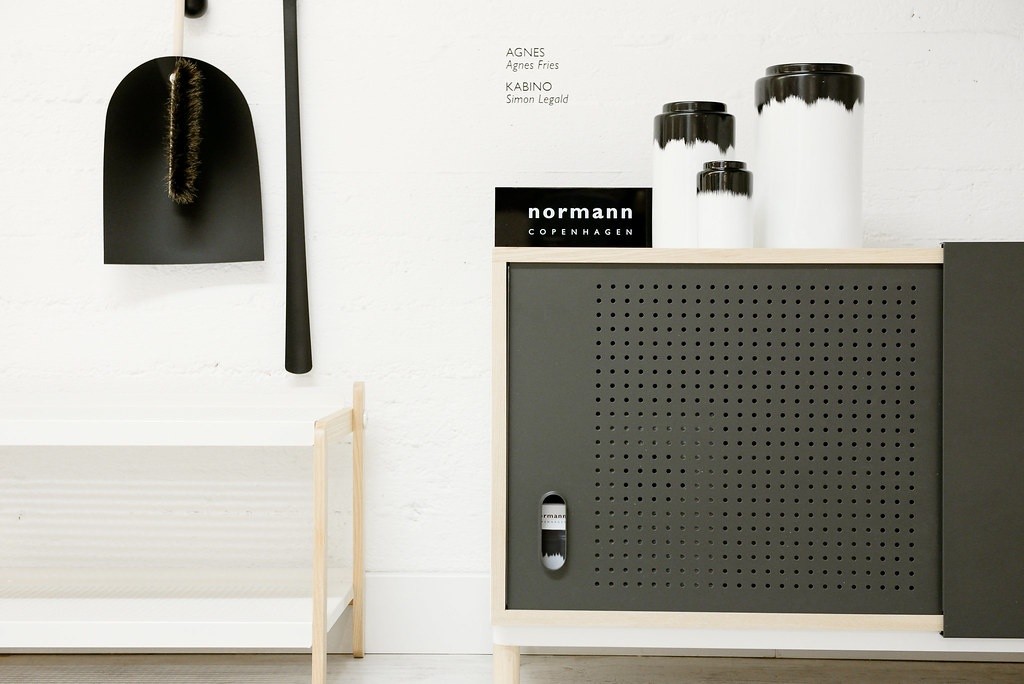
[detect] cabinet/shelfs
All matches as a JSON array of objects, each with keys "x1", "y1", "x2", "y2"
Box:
[{"x1": 0, "y1": 381, "x2": 368, "y2": 684}]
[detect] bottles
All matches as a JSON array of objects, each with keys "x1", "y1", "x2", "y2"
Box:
[
  {"x1": 755, "y1": 64, "x2": 865, "y2": 250},
  {"x1": 695, "y1": 158, "x2": 753, "y2": 249},
  {"x1": 653, "y1": 101, "x2": 736, "y2": 246}
]
